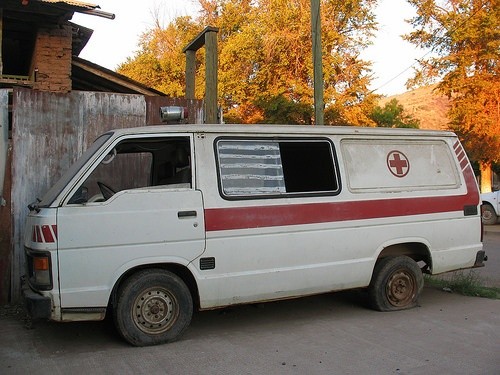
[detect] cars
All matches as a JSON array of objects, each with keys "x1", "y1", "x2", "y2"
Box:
[{"x1": 480, "y1": 189, "x2": 500, "y2": 225}]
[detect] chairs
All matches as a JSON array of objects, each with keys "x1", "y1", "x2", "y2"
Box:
[
  {"x1": 315, "y1": 171, "x2": 336, "y2": 191},
  {"x1": 176, "y1": 144, "x2": 193, "y2": 185}
]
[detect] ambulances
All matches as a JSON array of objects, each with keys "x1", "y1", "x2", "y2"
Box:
[{"x1": 17, "y1": 104, "x2": 489, "y2": 347}]
